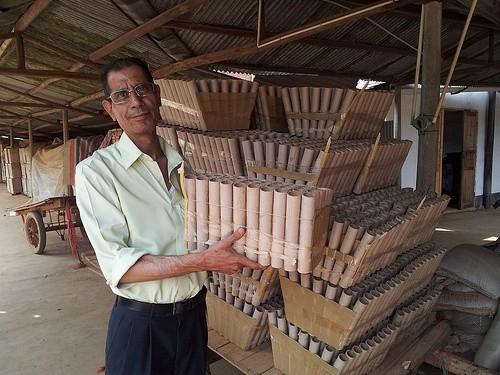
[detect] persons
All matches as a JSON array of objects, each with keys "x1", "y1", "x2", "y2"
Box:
[{"x1": 74, "y1": 57, "x2": 269, "y2": 375}]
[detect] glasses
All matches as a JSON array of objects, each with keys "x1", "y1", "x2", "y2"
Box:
[{"x1": 104, "y1": 81, "x2": 156, "y2": 104}]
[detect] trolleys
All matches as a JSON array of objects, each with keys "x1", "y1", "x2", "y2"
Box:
[{"x1": 6, "y1": 195, "x2": 87, "y2": 254}]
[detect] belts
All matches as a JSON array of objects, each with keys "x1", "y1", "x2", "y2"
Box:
[{"x1": 116, "y1": 285, "x2": 209, "y2": 315}]
